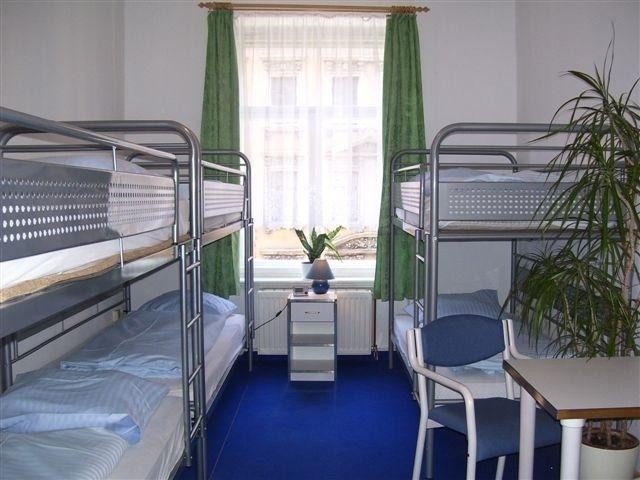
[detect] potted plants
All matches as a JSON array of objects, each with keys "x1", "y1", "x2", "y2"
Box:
[
  {"x1": 293, "y1": 225, "x2": 347, "y2": 282},
  {"x1": 489, "y1": 82, "x2": 640, "y2": 480}
]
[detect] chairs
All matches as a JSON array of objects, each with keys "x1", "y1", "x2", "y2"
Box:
[{"x1": 407, "y1": 315, "x2": 562, "y2": 480}]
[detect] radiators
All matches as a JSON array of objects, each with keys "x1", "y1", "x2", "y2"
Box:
[{"x1": 254, "y1": 287, "x2": 374, "y2": 355}]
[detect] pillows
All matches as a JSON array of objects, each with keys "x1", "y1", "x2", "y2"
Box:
[{"x1": 402, "y1": 289, "x2": 514, "y2": 321}]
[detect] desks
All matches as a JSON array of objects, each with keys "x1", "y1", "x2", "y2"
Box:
[{"x1": 498, "y1": 355, "x2": 640, "y2": 480}]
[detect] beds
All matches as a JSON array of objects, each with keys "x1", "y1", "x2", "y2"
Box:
[
  {"x1": 0, "y1": 106, "x2": 254, "y2": 480},
  {"x1": 389, "y1": 123, "x2": 640, "y2": 480}
]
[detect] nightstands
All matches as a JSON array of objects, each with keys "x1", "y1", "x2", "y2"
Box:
[{"x1": 285, "y1": 290, "x2": 339, "y2": 385}]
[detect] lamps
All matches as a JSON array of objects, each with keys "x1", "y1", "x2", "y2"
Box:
[{"x1": 306, "y1": 257, "x2": 335, "y2": 295}]
